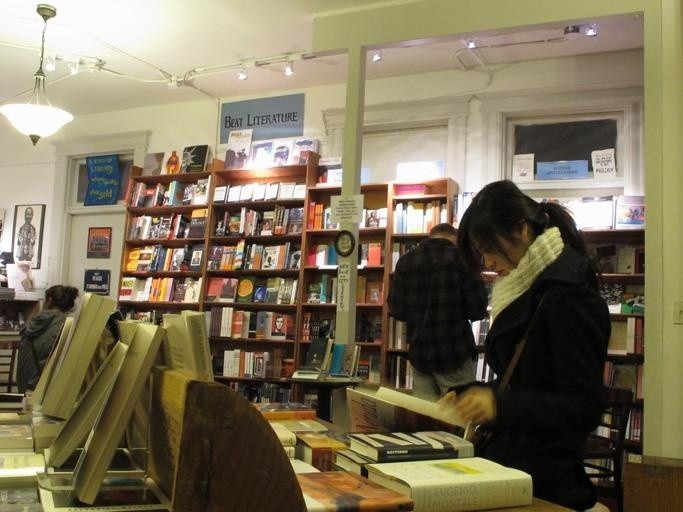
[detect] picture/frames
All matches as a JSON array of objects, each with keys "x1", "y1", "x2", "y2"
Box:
[
  {"x1": 9, "y1": 203, "x2": 46, "y2": 269},
  {"x1": 83, "y1": 268, "x2": 111, "y2": 296},
  {"x1": 86, "y1": 227, "x2": 111, "y2": 261}
]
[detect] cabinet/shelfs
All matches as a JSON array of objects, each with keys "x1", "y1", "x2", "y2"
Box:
[
  {"x1": 0, "y1": 298, "x2": 45, "y2": 336},
  {"x1": 114, "y1": 157, "x2": 211, "y2": 324},
  {"x1": 384, "y1": 177, "x2": 458, "y2": 396},
  {"x1": 292, "y1": 182, "x2": 387, "y2": 411},
  {"x1": 200, "y1": 148, "x2": 318, "y2": 411},
  {"x1": 0, "y1": 350, "x2": 421, "y2": 510},
  {"x1": 449, "y1": 188, "x2": 646, "y2": 453}
]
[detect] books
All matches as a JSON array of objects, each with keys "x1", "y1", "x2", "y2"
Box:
[
  {"x1": 612, "y1": 195, "x2": 646, "y2": 229},
  {"x1": 394, "y1": 185, "x2": 430, "y2": 196},
  {"x1": 203, "y1": 308, "x2": 294, "y2": 339},
  {"x1": 119, "y1": 277, "x2": 202, "y2": 301},
  {"x1": 224, "y1": 129, "x2": 253, "y2": 169},
  {"x1": 607, "y1": 317, "x2": 643, "y2": 355},
  {"x1": 293, "y1": 338, "x2": 375, "y2": 379},
  {"x1": 0, "y1": 348, "x2": 20, "y2": 395},
  {"x1": 475, "y1": 353, "x2": 498, "y2": 382},
  {"x1": 179, "y1": 145, "x2": 211, "y2": 172},
  {"x1": 347, "y1": 386, "x2": 468, "y2": 439},
  {"x1": 617, "y1": 246, "x2": 644, "y2": 274},
  {"x1": 292, "y1": 139, "x2": 318, "y2": 165},
  {"x1": 129, "y1": 208, "x2": 208, "y2": 239},
  {"x1": 388, "y1": 317, "x2": 409, "y2": 351},
  {"x1": 43, "y1": 339, "x2": 129, "y2": 470},
  {"x1": 454, "y1": 193, "x2": 475, "y2": 229},
  {"x1": 583, "y1": 405, "x2": 618, "y2": 489},
  {"x1": 33, "y1": 293, "x2": 117, "y2": 421},
  {"x1": 625, "y1": 408, "x2": 642, "y2": 444},
  {"x1": 163, "y1": 311, "x2": 214, "y2": 382},
  {"x1": 1, "y1": 453, "x2": 45, "y2": 488},
  {"x1": 130, "y1": 179, "x2": 209, "y2": 206},
  {"x1": 358, "y1": 242, "x2": 385, "y2": 267},
  {"x1": 204, "y1": 276, "x2": 298, "y2": 304},
  {"x1": 1, "y1": 412, "x2": 32, "y2": 424},
  {"x1": 222, "y1": 380, "x2": 326, "y2": 418},
  {"x1": 78, "y1": 324, "x2": 167, "y2": 506},
  {"x1": 356, "y1": 275, "x2": 383, "y2": 304},
  {"x1": 213, "y1": 205, "x2": 304, "y2": 236},
  {"x1": 360, "y1": 207, "x2": 388, "y2": 228},
  {"x1": 0, "y1": 424, "x2": 34, "y2": 449},
  {"x1": 1, "y1": 287, "x2": 15, "y2": 301},
  {"x1": 604, "y1": 361, "x2": 613, "y2": 388},
  {"x1": 307, "y1": 202, "x2": 340, "y2": 229},
  {"x1": 253, "y1": 142, "x2": 272, "y2": 165},
  {"x1": 306, "y1": 273, "x2": 338, "y2": 303},
  {"x1": 366, "y1": 457, "x2": 533, "y2": 511},
  {"x1": 309, "y1": 243, "x2": 337, "y2": 267},
  {"x1": 392, "y1": 201, "x2": 446, "y2": 234},
  {"x1": 334, "y1": 449, "x2": 366, "y2": 475},
  {"x1": 470, "y1": 320, "x2": 490, "y2": 346},
  {"x1": 349, "y1": 430, "x2": 475, "y2": 463},
  {"x1": 273, "y1": 147, "x2": 288, "y2": 165},
  {"x1": 121, "y1": 309, "x2": 163, "y2": 323},
  {"x1": 212, "y1": 182, "x2": 306, "y2": 203},
  {"x1": 209, "y1": 240, "x2": 301, "y2": 270},
  {"x1": 387, "y1": 354, "x2": 414, "y2": 389},
  {"x1": 316, "y1": 157, "x2": 343, "y2": 185},
  {"x1": 392, "y1": 242, "x2": 421, "y2": 272},
  {"x1": 125, "y1": 245, "x2": 204, "y2": 272},
  {"x1": 211, "y1": 348, "x2": 295, "y2": 379},
  {"x1": 269, "y1": 417, "x2": 348, "y2": 473}
]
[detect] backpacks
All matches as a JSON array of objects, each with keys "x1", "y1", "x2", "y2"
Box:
[{"x1": 475, "y1": 294, "x2": 546, "y2": 466}]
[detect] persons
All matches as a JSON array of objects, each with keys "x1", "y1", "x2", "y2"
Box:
[
  {"x1": 387, "y1": 222, "x2": 486, "y2": 403},
  {"x1": 16, "y1": 286, "x2": 81, "y2": 393},
  {"x1": 457, "y1": 179, "x2": 611, "y2": 512},
  {"x1": 18, "y1": 208, "x2": 35, "y2": 262},
  {"x1": 273, "y1": 315, "x2": 284, "y2": 337}
]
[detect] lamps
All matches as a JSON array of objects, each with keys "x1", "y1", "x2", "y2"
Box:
[
  {"x1": 43, "y1": 55, "x2": 57, "y2": 74},
  {"x1": 68, "y1": 58, "x2": 79, "y2": 75},
  {"x1": 370, "y1": 48, "x2": 382, "y2": 62},
  {"x1": 583, "y1": 23, "x2": 600, "y2": 40},
  {"x1": 282, "y1": 59, "x2": 291, "y2": 75},
  {"x1": 0, "y1": 3, "x2": 85, "y2": 147},
  {"x1": 466, "y1": 40, "x2": 479, "y2": 50},
  {"x1": 235, "y1": 65, "x2": 247, "y2": 81}
]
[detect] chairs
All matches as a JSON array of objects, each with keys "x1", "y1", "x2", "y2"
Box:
[
  {"x1": 574, "y1": 376, "x2": 631, "y2": 506},
  {"x1": 0, "y1": 339, "x2": 21, "y2": 393}
]
